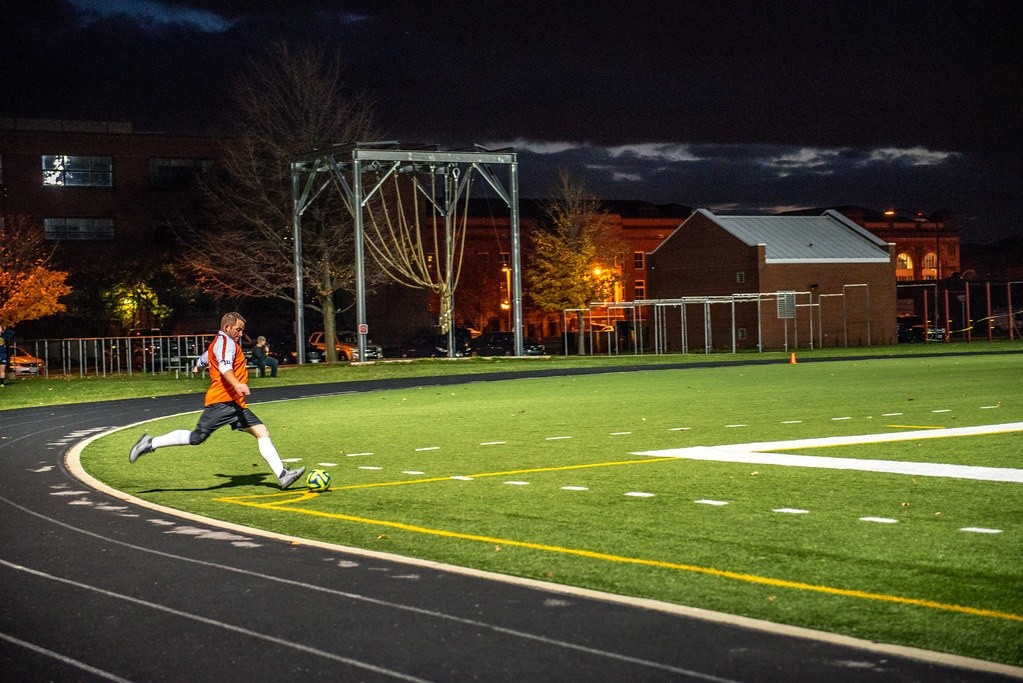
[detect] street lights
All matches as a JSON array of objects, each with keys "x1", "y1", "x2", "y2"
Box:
[{"x1": 501, "y1": 266, "x2": 510, "y2": 332}]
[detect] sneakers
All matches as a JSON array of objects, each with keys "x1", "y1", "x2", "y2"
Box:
[
  {"x1": 278, "y1": 468, "x2": 304, "y2": 489},
  {"x1": 128, "y1": 433, "x2": 150, "y2": 464}
]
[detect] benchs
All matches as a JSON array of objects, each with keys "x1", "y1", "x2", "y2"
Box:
[
  {"x1": 165, "y1": 365, "x2": 195, "y2": 380},
  {"x1": 199, "y1": 366, "x2": 267, "y2": 378}
]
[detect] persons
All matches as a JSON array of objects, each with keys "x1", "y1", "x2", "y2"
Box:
[
  {"x1": 129, "y1": 312, "x2": 305, "y2": 490},
  {"x1": 251, "y1": 336, "x2": 278, "y2": 378},
  {"x1": 0, "y1": 319, "x2": 15, "y2": 387}
]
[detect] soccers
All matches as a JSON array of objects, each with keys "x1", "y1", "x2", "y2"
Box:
[{"x1": 306, "y1": 468, "x2": 331, "y2": 493}]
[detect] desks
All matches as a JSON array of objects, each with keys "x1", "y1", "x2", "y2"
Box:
[{"x1": 174, "y1": 355, "x2": 252, "y2": 378}]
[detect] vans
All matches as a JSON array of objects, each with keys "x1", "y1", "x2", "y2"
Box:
[{"x1": 990, "y1": 308, "x2": 1023, "y2": 334}]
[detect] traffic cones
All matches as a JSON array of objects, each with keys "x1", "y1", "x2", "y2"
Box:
[{"x1": 788, "y1": 352, "x2": 797, "y2": 364}]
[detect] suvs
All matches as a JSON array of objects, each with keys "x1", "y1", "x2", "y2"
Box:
[
  {"x1": 308, "y1": 331, "x2": 384, "y2": 361},
  {"x1": 896, "y1": 313, "x2": 947, "y2": 343}
]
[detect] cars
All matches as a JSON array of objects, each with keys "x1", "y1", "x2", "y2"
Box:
[
  {"x1": 472, "y1": 331, "x2": 547, "y2": 356},
  {"x1": 99, "y1": 328, "x2": 290, "y2": 373},
  {"x1": 273, "y1": 333, "x2": 325, "y2": 363},
  {"x1": 10, "y1": 346, "x2": 44, "y2": 376},
  {"x1": 382, "y1": 327, "x2": 476, "y2": 358}
]
